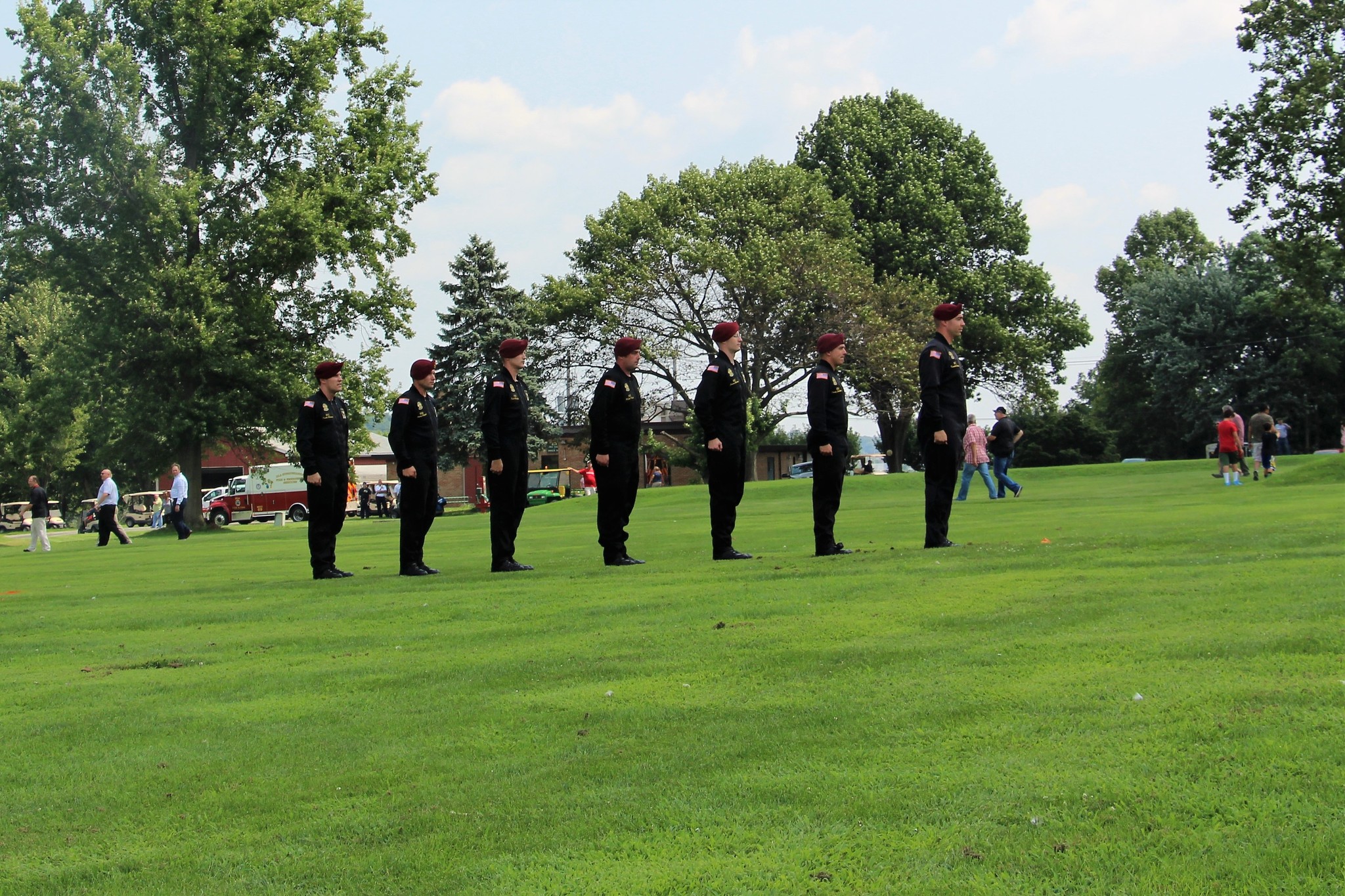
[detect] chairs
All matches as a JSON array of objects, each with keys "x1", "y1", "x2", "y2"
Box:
[
  {"x1": 13, "y1": 513, "x2": 21, "y2": 521},
  {"x1": 557, "y1": 486, "x2": 566, "y2": 498},
  {"x1": 133, "y1": 504, "x2": 146, "y2": 514},
  {"x1": 5, "y1": 514, "x2": 14, "y2": 522}
]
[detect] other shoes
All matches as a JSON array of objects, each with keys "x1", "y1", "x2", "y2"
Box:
[
  {"x1": 1014, "y1": 486, "x2": 1022, "y2": 497},
  {"x1": 23, "y1": 549, "x2": 30, "y2": 552},
  {"x1": 1211, "y1": 467, "x2": 1275, "y2": 486},
  {"x1": 187, "y1": 530, "x2": 192, "y2": 539}
]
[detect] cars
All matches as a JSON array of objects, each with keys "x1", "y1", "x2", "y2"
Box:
[
  {"x1": 1313, "y1": 448, "x2": 1345, "y2": 454},
  {"x1": 1121, "y1": 458, "x2": 1152, "y2": 462},
  {"x1": 782, "y1": 461, "x2": 813, "y2": 480}
]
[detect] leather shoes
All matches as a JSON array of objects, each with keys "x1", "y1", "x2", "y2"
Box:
[
  {"x1": 836, "y1": 550, "x2": 852, "y2": 554},
  {"x1": 400, "y1": 563, "x2": 428, "y2": 576},
  {"x1": 423, "y1": 568, "x2": 440, "y2": 574},
  {"x1": 722, "y1": 550, "x2": 752, "y2": 559},
  {"x1": 512, "y1": 561, "x2": 534, "y2": 570},
  {"x1": 617, "y1": 558, "x2": 635, "y2": 565},
  {"x1": 331, "y1": 569, "x2": 353, "y2": 577},
  {"x1": 626, "y1": 557, "x2": 645, "y2": 564},
  {"x1": 496, "y1": 560, "x2": 525, "y2": 572},
  {"x1": 313, "y1": 569, "x2": 343, "y2": 579}
]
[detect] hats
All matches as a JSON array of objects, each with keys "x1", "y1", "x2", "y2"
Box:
[
  {"x1": 993, "y1": 406, "x2": 1006, "y2": 414},
  {"x1": 614, "y1": 338, "x2": 642, "y2": 356},
  {"x1": 816, "y1": 333, "x2": 845, "y2": 353},
  {"x1": 499, "y1": 339, "x2": 528, "y2": 359},
  {"x1": 410, "y1": 359, "x2": 436, "y2": 380},
  {"x1": 363, "y1": 482, "x2": 366, "y2": 485},
  {"x1": 314, "y1": 362, "x2": 344, "y2": 379},
  {"x1": 933, "y1": 303, "x2": 962, "y2": 321},
  {"x1": 711, "y1": 322, "x2": 740, "y2": 342}
]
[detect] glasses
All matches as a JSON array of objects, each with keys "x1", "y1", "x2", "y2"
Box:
[
  {"x1": 733, "y1": 333, "x2": 741, "y2": 337},
  {"x1": 101, "y1": 473, "x2": 108, "y2": 475}
]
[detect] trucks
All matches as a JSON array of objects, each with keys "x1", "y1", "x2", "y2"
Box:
[{"x1": 202, "y1": 457, "x2": 357, "y2": 526}]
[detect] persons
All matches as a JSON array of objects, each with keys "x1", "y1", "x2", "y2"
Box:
[
  {"x1": 81, "y1": 463, "x2": 174, "y2": 547},
  {"x1": 861, "y1": 460, "x2": 873, "y2": 475},
  {"x1": 588, "y1": 337, "x2": 645, "y2": 566},
  {"x1": 479, "y1": 339, "x2": 536, "y2": 572},
  {"x1": 952, "y1": 405, "x2": 1024, "y2": 500},
  {"x1": 917, "y1": 304, "x2": 968, "y2": 549},
  {"x1": 359, "y1": 478, "x2": 401, "y2": 520},
  {"x1": 695, "y1": 322, "x2": 752, "y2": 561},
  {"x1": 387, "y1": 358, "x2": 442, "y2": 577},
  {"x1": 567, "y1": 459, "x2": 598, "y2": 496},
  {"x1": 649, "y1": 467, "x2": 663, "y2": 490},
  {"x1": 20, "y1": 476, "x2": 51, "y2": 552},
  {"x1": 806, "y1": 334, "x2": 853, "y2": 555},
  {"x1": 1209, "y1": 401, "x2": 1345, "y2": 487},
  {"x1": 294, "y1": 360, "x2": 355, "y2": 578},
  {"x1": 170, "y1": 464, "x2": 194, "y2": 540}
]
